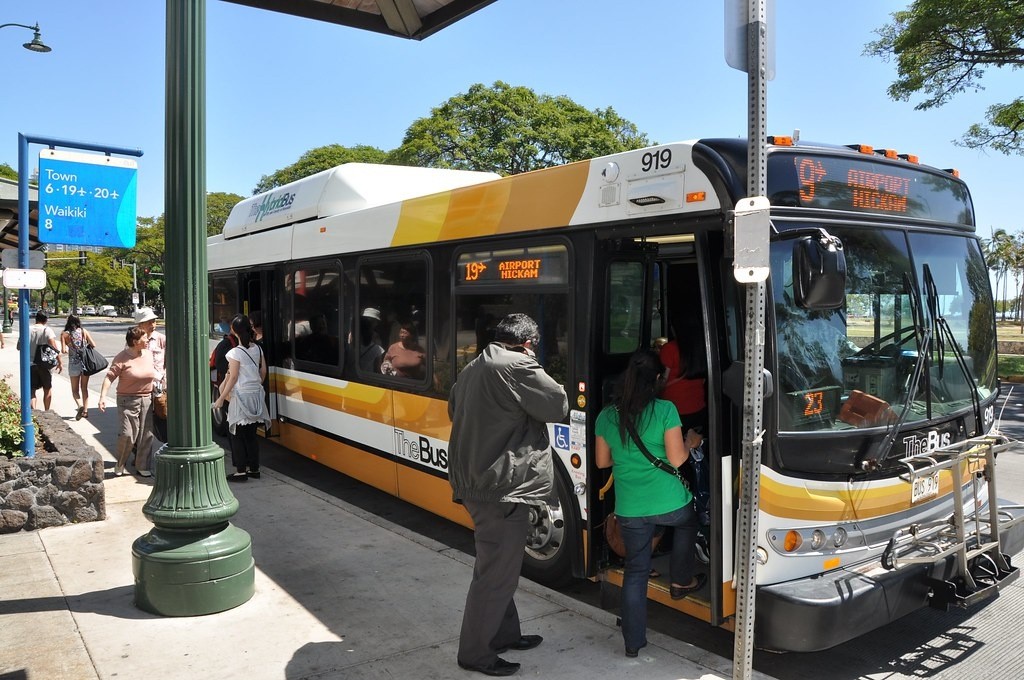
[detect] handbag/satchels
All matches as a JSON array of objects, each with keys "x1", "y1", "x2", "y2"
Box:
[
  {"x1": 153, "y1": 380, "x2": 167, "y2": 420},
  {"x1": 77, "y1": 346, "x2": 109, "y2": 375},
  {"x1": 603, "y1": 512, "x2": 666, "y2": 558},
  {"x1": 693, "y1": 488, "x2": 710, "y2": 527},
  {"x1": 219, "y1": 368, "x2": 233, "y2": 403},
  {"x1": 34, "y1": 328, "x2": 60, "y2": 371}
]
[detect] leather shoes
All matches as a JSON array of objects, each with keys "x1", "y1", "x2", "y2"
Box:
[
  {"x1": 458, "y1": 655, "x2": 521, "y2": 676},
  {"x1": 495, "y1": 635, "x2": 543, "y2": 654}
]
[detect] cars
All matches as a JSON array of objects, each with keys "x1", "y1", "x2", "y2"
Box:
[
  {"x1": 28, "y1": 307, "x2": 38, "y2": 317},
  {"x1": 46, "y1": 304, "x2": 117, "y2": 318}
]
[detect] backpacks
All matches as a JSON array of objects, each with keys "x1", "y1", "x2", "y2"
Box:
[{"x1": 209, "y1": 336, "x2": 237, "y2": 387}]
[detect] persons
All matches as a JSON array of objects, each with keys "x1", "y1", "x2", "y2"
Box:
[
  {"x1": 8, "y1": 305, "x2": 16, "y2": 325},
  {"x1": 653, "y1": 300, "x2": 710, "y2": 570},
  {"x1": 595, "y1": 353, "x2": 705, "y2": 659},
  {"x1": 98, "y1": 308, "x2": 166, "y2": 478},
  {"x1": 0, "y1": 322, "x2": 5, "y2": 352},
  {"x1": 16, "y1": 310, "x2": 64, "y2": 411},
  {"x1": 209, "y1": 306, "x2": 269, "y2": 484},
  {"x1": 788, "y1": 309, "x2": 864, "y2": 387},
  {"x1": 352, "y1": 308, "x2": 438, "y2": 393},
  {"x1": 448, "y1": 313, "x2": 569, "y2": 677},
  {"x1": 60, "y1": 314, "x2": 96, "y2": 420}
]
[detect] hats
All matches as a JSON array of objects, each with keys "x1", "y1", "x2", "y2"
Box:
[
  {"x1": 360, "y1": 307, "x2": 382, "y2": 321},
  {"x1": 135, "y1": 307, "x2": 158, "y2": 324}
]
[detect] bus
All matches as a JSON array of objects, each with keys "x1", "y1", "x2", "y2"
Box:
[{"x1": 206, "y1": 136, "x2": 1024, "y2": 653}]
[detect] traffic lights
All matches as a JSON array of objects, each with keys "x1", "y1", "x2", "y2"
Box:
[
  {"x1": 144, "y1": 267, "x2": 149, "y2": 277},
  {"x1": 119, "y1": 260, "x2": 122, "y2": 269},
  {"x1": 109, "y1": 261, "x2": 114, "y2": 269}
]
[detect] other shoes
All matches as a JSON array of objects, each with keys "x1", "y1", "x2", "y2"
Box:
[
  {"x1": 114, "y1": 462, "x2": 124, "y2": 476},
  {"x1": 82, "y1": 412, "x2": 88, "y2": 418},
  {"x1": 76, "y1": 406, "x2": 84, "y2": 421},
  {"x1": 694, "y1": 531, "x2": 710, "y2": 567},
  {"x1": 137, "y1": 469, "x2": 151, "y2": 477},
  {"x1": 625, "y1": 640, "x2": 647, "y2": 658},
  {"x1": 649, "y1": 569, "x2": 661, "y2": 577},
  {"x1": 226, "y1": 470, "x2": 260, "y2": 482},
  {"x1": 670, "y1": 573, "x2": 707, "y2": 601}
]
